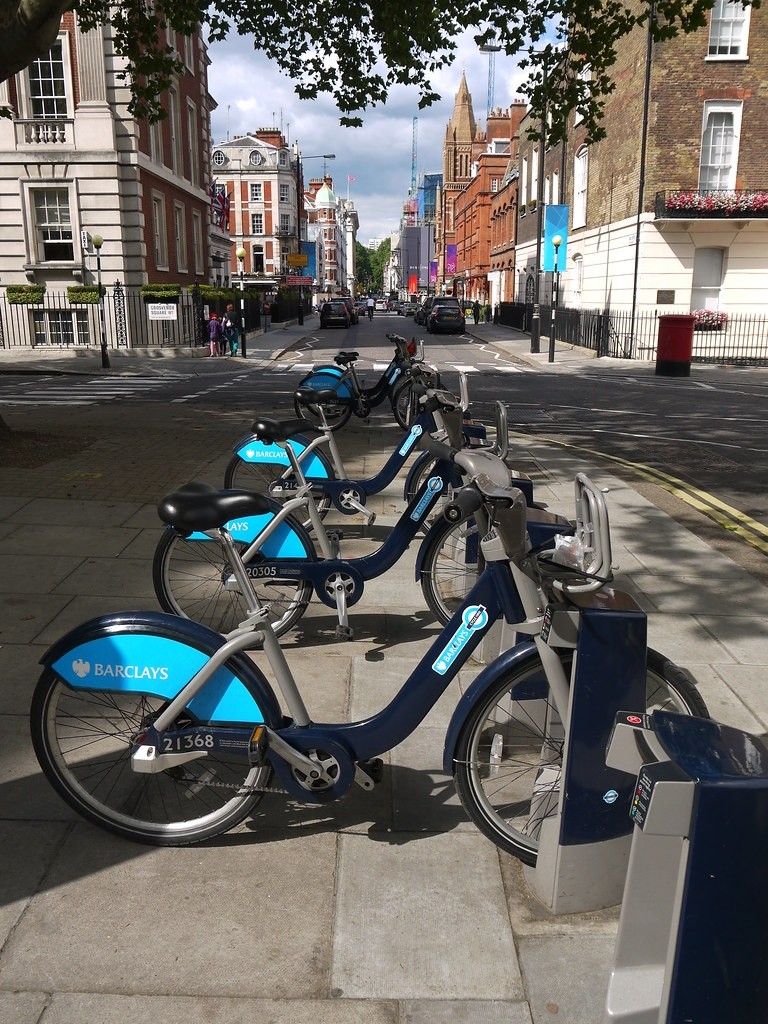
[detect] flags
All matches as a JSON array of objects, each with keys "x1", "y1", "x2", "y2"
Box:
[
  {"x1": 349, "y1": 176, "x2": 355, "y2": 181},
  {"x1": 209, "y1": 182, "x2": 230, "y2": 235}
]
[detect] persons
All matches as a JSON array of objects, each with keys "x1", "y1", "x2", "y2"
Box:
[
  {"x1": 471, "y1": 300, "x2": 482, "y2": 324},
  {"x1": 367, "y1": 297, "x2": 392, "y2": 319},
  {"x1": 313, "y1": 297, "x2": 331, "y2": 315},
  {"x1": 208, "y1": 304, "x2": 240, "y2": 356}
]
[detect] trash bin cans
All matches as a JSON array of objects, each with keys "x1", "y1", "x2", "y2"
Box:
[{"x1": 655, "y1": 314, "x2": 698, "y2": 377}]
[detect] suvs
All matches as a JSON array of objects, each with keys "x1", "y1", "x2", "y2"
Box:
[{"x1": 327, "y1": 297, "x2": 359, "y2": 325}]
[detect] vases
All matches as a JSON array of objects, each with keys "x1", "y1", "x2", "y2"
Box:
[{"x1": 695, "y1": 324, "x2": 722, "y2": 330}]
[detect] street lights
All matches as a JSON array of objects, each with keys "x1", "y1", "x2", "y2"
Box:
[
  {"x1": 93, "y1": 233, "x2": 112, "y2": 369},
  {"x1": 479, "y1": 44, "x2": 550, "y2": 353},
  {"x1": 296, "y1": 154, "x2": 337, "y2": 326},
  {"x1": 236, "y1": 247, "x2": 247, "y2": 358},
  {"x1": 549, "y1": 236, "x2": 562, "y2": 363},
  {"x1": 417, "y1": 186, "x2": 446, "y2": 297}
]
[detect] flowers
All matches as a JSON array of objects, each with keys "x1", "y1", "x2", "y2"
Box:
[
  {"x1": 665, "y1": 192, "x2": 768, "y2": 213},
  {"x1": 691, "y1": 309, "x2": 728, "y2": 326}
]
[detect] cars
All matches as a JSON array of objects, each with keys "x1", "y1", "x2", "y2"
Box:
[
  {"x1": 318, "y1": 301, "x2": 351, "y2": 329},
  {"x1": 388, "y1": 300, "x2": 398, "y2": 311},
  {"x1": 374, "y1": 299, "x2": 387, "y2": 311},
  {"x1": 426, "y1": 304, "x2": 466, "y2": 335},
  {"x1": 356, "y1": 296, "x2": 477, "y2": 327}
]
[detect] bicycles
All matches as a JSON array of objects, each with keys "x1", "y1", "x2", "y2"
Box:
[
  {"x1": 150, "y1": 383, "x2": 580, "y2": 649},
  {"x1": 222, "y1": 384, "x2": 547, "y2": 535},
  {"x1": 30, "y1": 439, "x2": 711, "y2": 870},
  {"x1": 294, "y1": 333, "x2": 469, "y2": 434}
]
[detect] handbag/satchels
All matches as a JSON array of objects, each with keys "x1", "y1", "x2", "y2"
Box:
[{"x1": 225, "y1": 313, "x2": 234, "y2": 327}]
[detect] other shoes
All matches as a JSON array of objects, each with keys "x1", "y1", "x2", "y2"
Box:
[
  {"x1": 210, "y1": 353, "x2": 214, "y2": 357},
  {"x1": 232, "y1": 352, "x2": 237, "y2": 357},
  {"x1": 215, "y1": 353, "x2": 219, "y2": 356}
]
